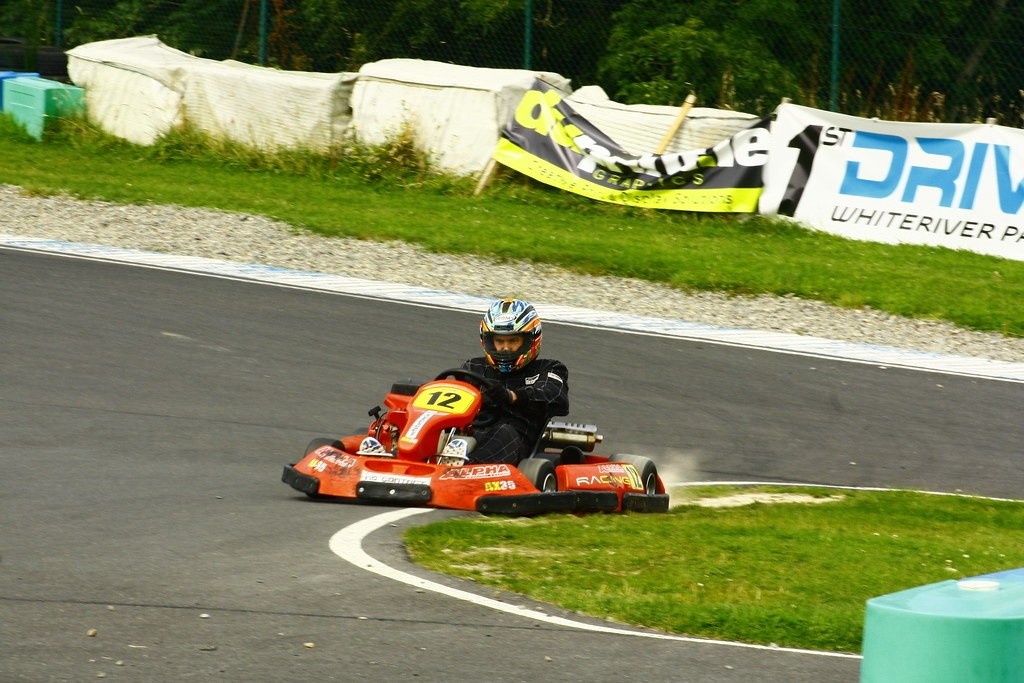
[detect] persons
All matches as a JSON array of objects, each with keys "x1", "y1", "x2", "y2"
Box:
[{"x1": 444, "y1": 296, "x2": 571, "y2": 467}]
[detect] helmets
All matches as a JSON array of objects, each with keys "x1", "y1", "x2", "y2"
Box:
[{"x1": 479, "y1": 298, "x2": 542, "y2": 377}]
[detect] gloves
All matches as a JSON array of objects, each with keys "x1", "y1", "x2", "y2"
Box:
[{"x1": 480, "y1": 379, "x2": 513, "y2": 406}]
